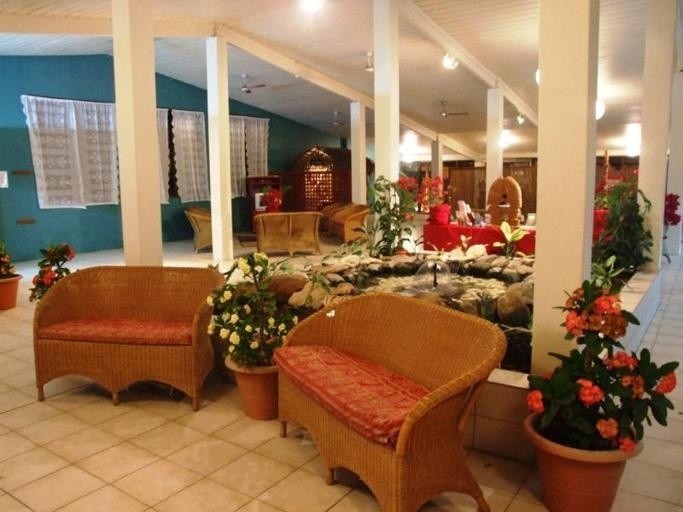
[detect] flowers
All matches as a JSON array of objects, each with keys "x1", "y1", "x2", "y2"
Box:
[
  {"x1": 200, "y1": 248, "x2": 304, "y2": 367},
  {"x1": 0, "y1": 240, "x2": 17, "y2": 278},
  {"x1": 373, "y1": 176, "x2": 419, "y2": 259},
  {"x1": 24, "y1": 240, "x2": 79, "y2": 304},
  {"x1": 520, "y1": 255, "x2": 679, "y2": 462}
]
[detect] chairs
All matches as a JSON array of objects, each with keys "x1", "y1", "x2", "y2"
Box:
[
  {"x1": 320, "y1": 200, "x2": 371, "y2": 247},
  {"x1": 182, "y1": 203, "x2": 211, "y2": 254}
]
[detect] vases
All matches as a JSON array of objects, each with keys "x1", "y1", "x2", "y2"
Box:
[
  {"x1": 223, "y1": 352, "x2": 282, "y2": 424},
  {"x1": 0, "y1": 273, "x2": 23, "y2": 312},
  {"x1": 522, "y1": 408, "x2": 642, "y2": 511}
]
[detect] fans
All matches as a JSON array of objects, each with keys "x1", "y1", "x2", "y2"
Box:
[
  {"x1": 359, "y1": 50, "x2": 375, "y2": 72},
  {"x1": 227, "y1": 73, "x2": 266, "y2": 96},
  {"x1": 437, "y1": 100, "x2": 470, "y2": 120},
  {"x1": 309, "y1": 110, "x2": 348, "y2": 128}
]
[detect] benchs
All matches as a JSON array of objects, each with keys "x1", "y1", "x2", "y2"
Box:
[
  {"x1": 250, "y1": 212, "x2": 324, "y2": 261},
  {"x1": 266, "y1": 292, "x2": 509, "y2": 511},
  {"x1": 28, "y1": 265, "x2": 228, "y2": 414}
]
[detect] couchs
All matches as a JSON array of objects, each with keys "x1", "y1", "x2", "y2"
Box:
[{"x1": 411, "y1": 172, "x2": 535, "y2": 257}]
